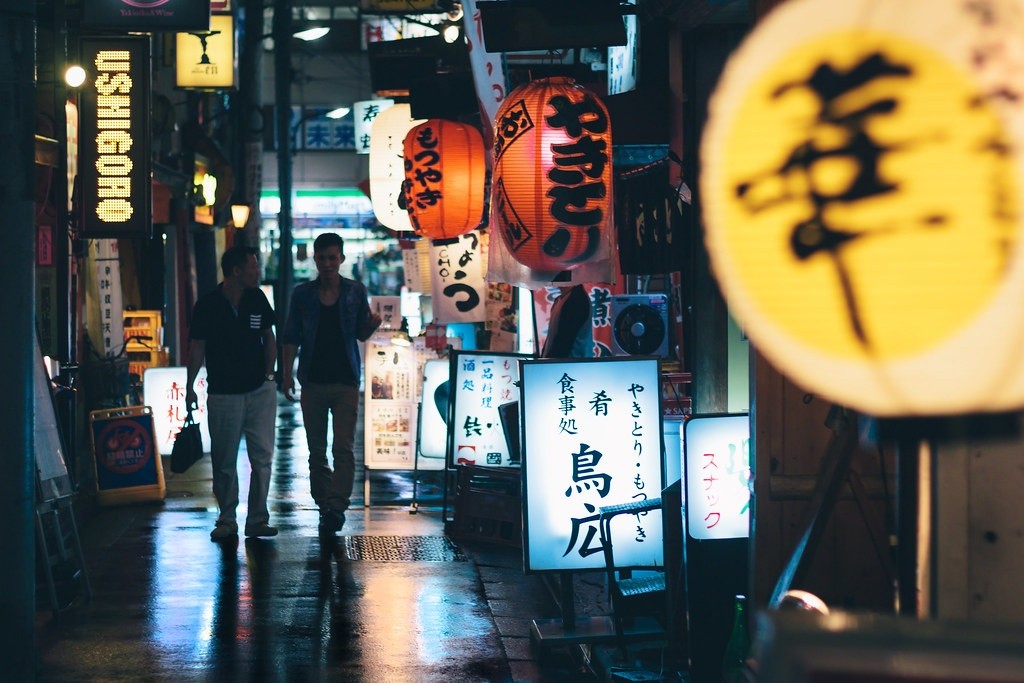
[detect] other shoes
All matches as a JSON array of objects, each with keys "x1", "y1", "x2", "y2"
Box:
[
  {"x1": 210, "y1": 522, "x2": 238, "y2": 538},
  {"x1": 319, "y1": 510, "x2": 342, "y2": 532},
  {"x1": 245, "y1": 522, "x2": 277, "y2": 536}
]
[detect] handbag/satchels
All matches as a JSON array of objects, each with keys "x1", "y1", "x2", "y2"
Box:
[{"x1": 169, "y1": 411, "x2": 204, "y2": 473}]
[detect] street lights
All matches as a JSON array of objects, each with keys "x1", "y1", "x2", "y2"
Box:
[{"x1": 274, "y1": 106, "x2": 351, "y2": 334}]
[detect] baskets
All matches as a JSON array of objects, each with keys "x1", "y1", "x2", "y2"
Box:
[{"x1": 79, "y1": 357, "x2": 129, "y2": 399}]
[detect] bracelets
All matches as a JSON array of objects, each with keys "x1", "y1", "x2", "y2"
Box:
[{"x1": 264, "y1": 369, "x2": 274, "y2": 380}]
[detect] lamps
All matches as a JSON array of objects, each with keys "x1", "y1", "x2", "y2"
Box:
[
  {"x1": 292, "y1": 9, "x2": 330, "y2": 43},
  {"x1": 231, "y1": 205, "x2": 250, "y2": 232},
  {"x1": 183, "y1": 173, "x2": 217, "y2": 206},
  {"x1": 400, "y1": 0, "x2": 464, "y2": 43}
]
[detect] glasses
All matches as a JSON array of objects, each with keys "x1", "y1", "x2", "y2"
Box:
[{"x1": 315, "y1": 254, "x2": 340, "y2": 261}]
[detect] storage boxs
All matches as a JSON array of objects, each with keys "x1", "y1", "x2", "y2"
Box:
[{"x1": 122, "y1": 309, "x2": 170, "y2": 382}]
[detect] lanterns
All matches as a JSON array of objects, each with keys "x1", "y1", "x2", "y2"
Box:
[
  {"x1": 369, "y1": 100, "x2": 428, "y2": 240},
  {"x1": 493, "y1": 66, "x2": 613, "y2": 282},
  {"x1": 404, "y1": 112, "x2": 484, "y2": 247},
  {"x1": 701, "y1": 1, "x2": 1024, "y2": 440}
]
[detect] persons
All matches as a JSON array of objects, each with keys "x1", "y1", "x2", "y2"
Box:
[
  {"x1": 282, "y1": 233, "x2": 382, "y2": 535},
  {"x1": 185, "y1": 246, "x2": 277, "y2": 538}
]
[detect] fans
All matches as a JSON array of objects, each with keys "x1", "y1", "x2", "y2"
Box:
[{"x1": 610, "y1": 293, "x2": 670, "y2": 362}]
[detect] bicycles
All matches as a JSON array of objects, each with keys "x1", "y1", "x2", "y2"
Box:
[{"x1": 82, "y1": 335, "x2": 153, "y2": 411}]
